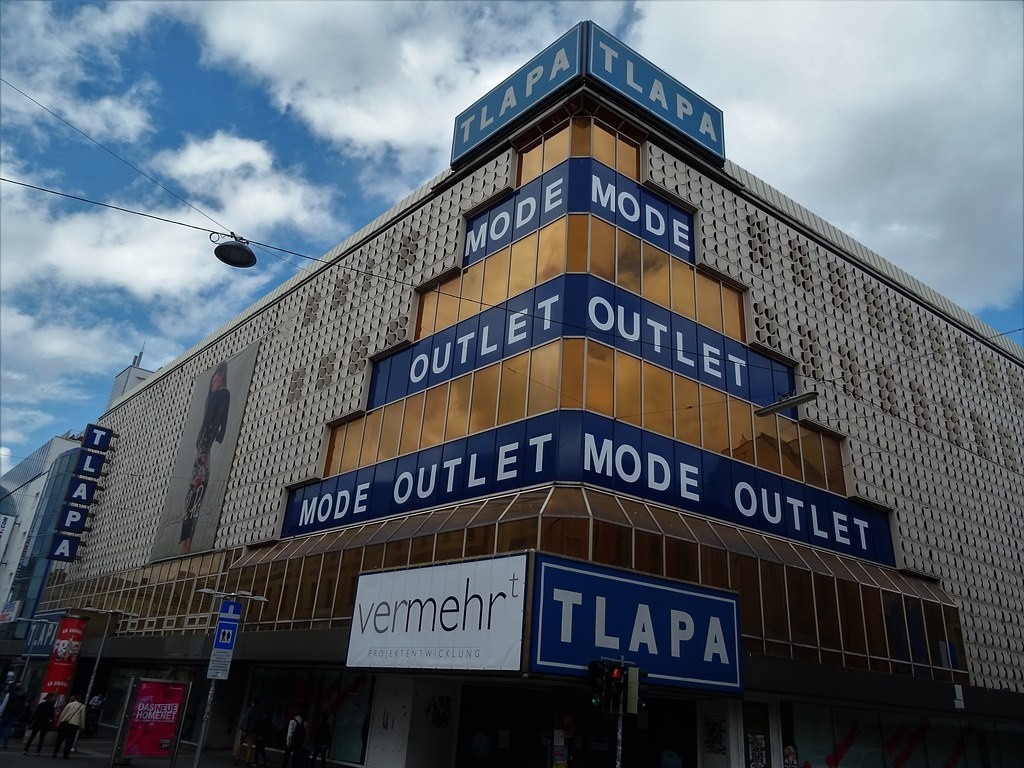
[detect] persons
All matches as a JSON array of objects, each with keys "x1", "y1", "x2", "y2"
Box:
[
  {"x1": 231, "y1": 696, "x2": 332, "y2": 768},
  {"x1": 1, "y1": 680, "x2": 107, "y2": 761},
  {"x1": 179, "y1": 361, "x2": 229, "y2": 554}
]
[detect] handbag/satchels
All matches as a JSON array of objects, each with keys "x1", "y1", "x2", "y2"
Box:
[{"x1": 57, "y1": 721, "x2": 71, "y2": 730}]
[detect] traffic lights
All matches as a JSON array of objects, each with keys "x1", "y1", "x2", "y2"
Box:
[
  {"x1": 605, "y1": 663, "x2": 624, "y2": 713},
  {"x1": 589, "y1": 661, "x2": 604, "y2": 710}
]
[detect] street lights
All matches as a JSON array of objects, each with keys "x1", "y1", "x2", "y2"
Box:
[
  {"x1": 192, "y1": 588, "x2": 270, "y2": 768},
  {"x1": 16, "y1": 618, "x2": 59, "y2": 685},
  {"x1": 70, "y1": 607, "x2": 138, "y2": 752}
]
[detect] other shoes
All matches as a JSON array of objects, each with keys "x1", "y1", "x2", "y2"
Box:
[
  {"x1": 9, "y1": 735, "x2": 18, "y2": 740},
  {"x1": 35, "y1": 753, "x2": 40, "y2": 756},
  {"x1": 262, "y1": 760, "x2": 267, "y2": 765},
  {"x1": 244, "y1": 760, "x2": 250, "y2": 764},
  {"x1": 252, "y1": 764, "x2": 258, "y2": 768},
  {"x1": 23, "y1": 751, "x2": 27, "y2": 755},
  {"x1": 52, "y1": 755, "x2": 57, "y2": 759},
  {"x1": 234, "y1": 760, "x2": 239, "y2": 766},
  {"x1": 63, "y1": 755, "x2": 70, "y2": 760}
]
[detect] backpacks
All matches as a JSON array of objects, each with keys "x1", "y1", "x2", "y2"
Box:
[{"x1": 291, "y1": 718, "x2": 306, "y2": 746}]
